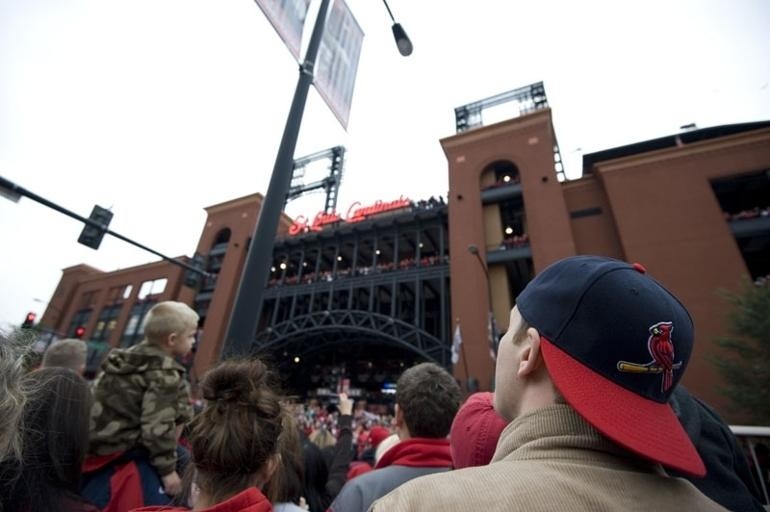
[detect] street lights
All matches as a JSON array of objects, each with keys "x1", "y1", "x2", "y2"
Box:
[
  {"x1": 370, "y1": 0, "x2": 413, "y2": 56},
  {"x1": 468, "y1": 244, "x2": 498, "y2": 359}
]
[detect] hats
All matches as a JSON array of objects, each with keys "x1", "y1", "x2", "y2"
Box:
[
  {"x1": 447, "y1": 390, "x2": 510, "y2": 470},
  {"x1": 513, "y1": 251, "x2": 708, "y2": 478}
]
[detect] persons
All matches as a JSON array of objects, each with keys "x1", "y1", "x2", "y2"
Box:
[
  {"x1": 366, "y1": 254, "x2": 729, "y2": 512},
  {"x1": 1, "y1": 301, "x2": 768, "y2": 511},
  {"x1": 266, "y1": 232, "x2": 530, "y2": 289}
]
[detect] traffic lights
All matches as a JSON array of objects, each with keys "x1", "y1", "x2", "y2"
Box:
[
  {"x1": 26, "y1": 312, "x2": 36, "y2": 322},
  {"x1": 75, "y1": 326, "x2": 85, "y2": 336}
]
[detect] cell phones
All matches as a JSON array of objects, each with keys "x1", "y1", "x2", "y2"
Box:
[{"x1": 329, "y1": 396, "x2": 340, "y2": 404}]
